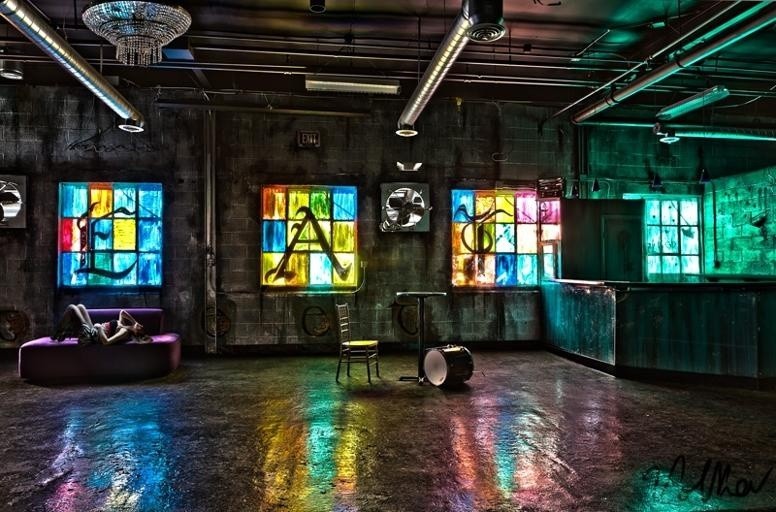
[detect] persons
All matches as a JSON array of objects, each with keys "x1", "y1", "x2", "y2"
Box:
[{"x1": 49, "y1": 303, "x2": 153, "y2": 351}]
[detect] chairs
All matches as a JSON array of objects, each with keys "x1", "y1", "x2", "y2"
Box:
[{"x1": 334, "y1": 303, "x2": 379, "y2": 383}]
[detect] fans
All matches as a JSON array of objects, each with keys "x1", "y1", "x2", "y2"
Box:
[
  {"x1": 0, "y1": 174, "x2": 29, "y2": 229},
  {"x1": 381, "y1": 181, "x2": 432, "y2": 233}
]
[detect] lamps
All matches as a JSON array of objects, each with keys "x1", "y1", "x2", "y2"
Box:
[
  {"x1": 592, "y1": 40, "x2": 600, "y2": 191},
  {"x1": 652, "y1": 79, "x2": 662, "y2": 187},
  {"x1": 524, "y1": 44, "x2": 531, "y2": 53},
  {"x1": 310, "y1": 0, "x2": 326, "y2": 14},
  {"x1": 699, "y1": 59, "x2": 709, "y2": 183},
  {"x1": 304, "y1": 75, "x2": 401, "y2": 96},
  {"x1": 658, "y1": 84, "x2": 730, "y2": 121},
  {"x1": 82, "y1": 0, "x2": 192, "y2": 66}
]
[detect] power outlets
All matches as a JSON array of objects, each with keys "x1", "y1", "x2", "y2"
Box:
[{"x1": 360, "y1": 261, "x2": 368, "y2": 268}]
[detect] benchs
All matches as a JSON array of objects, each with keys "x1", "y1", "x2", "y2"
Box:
[{"x1": 18, "y1": 307, "x2": 181, "y2": 383}]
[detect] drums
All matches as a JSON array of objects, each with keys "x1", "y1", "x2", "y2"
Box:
[{"x1": 424, "y1": 346, "x2": 474, "y2": 387}]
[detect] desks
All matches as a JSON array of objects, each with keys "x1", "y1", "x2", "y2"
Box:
[{"x1": 396, "y1": 292, "x2": 447, "y2": 385}]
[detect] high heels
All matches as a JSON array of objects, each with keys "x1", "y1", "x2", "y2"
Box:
[{"x1": 51, "y1": 328, "x2": 73, "y2": 342}]
